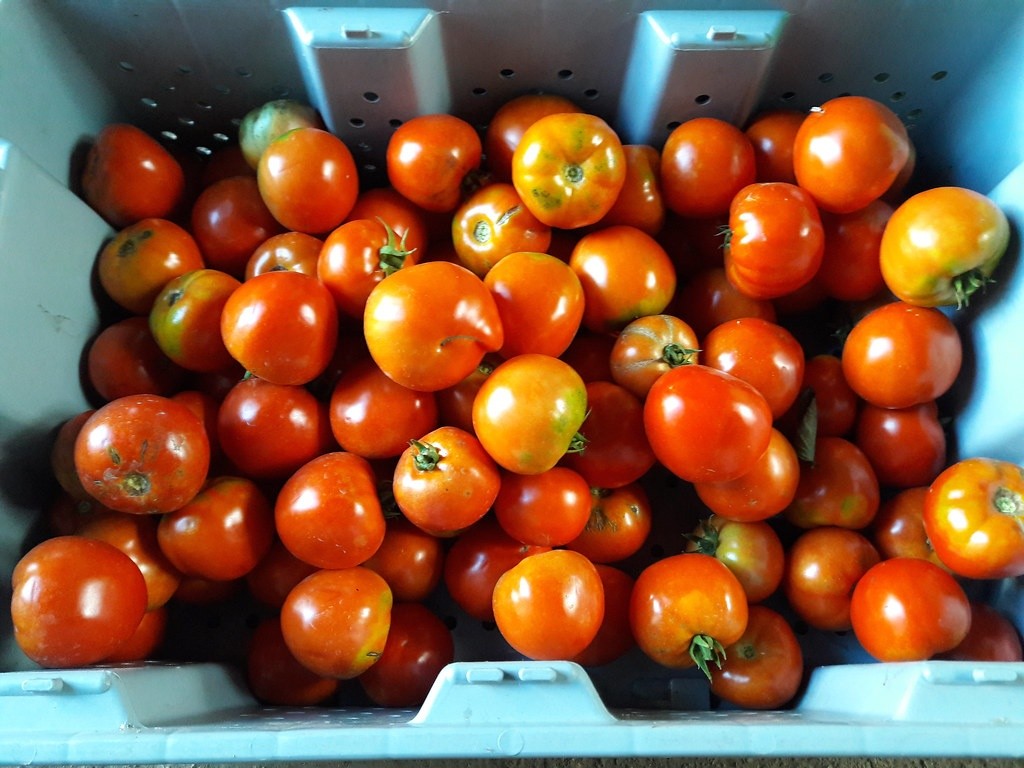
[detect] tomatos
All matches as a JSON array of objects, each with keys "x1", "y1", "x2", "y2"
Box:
[{"x1": 12, "y1": 93, "x2": 1024, "y2": 708}]
